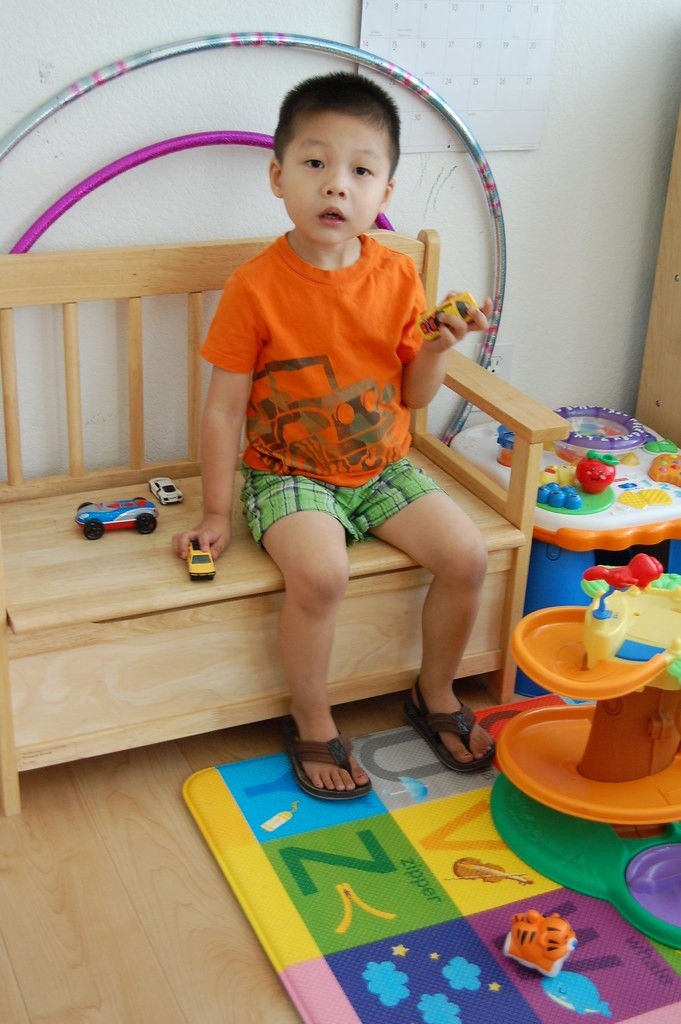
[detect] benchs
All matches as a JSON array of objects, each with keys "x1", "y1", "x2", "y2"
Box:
[{"x1": 0, "y1": 229, "x2": 572, "y2": 818}]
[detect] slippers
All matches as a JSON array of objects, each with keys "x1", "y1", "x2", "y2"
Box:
[
  {"x1": 403, "y1": 674, "x2": 496, "y2": 772},
  {"x1": 281, "y1": 716, "x2": 373, "y2": 800}
]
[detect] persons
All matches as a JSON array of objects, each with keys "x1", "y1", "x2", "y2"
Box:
[{"x1": 171, "y1": 69, "x2": 498, "y2": 803}]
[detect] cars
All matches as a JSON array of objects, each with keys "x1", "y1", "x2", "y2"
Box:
[
  {"x1": 148, "y1": 476, "x2": 183, "y2": 505},
  {"x1": 74, "y1": 496, "x2": 159, "y2": 540},
  {"x1": 414, "y1": 292, "x2": 481, "y2": 342},
  {"x1": 185, "y1": 539, "x2": 216, "y2": 582}
]
